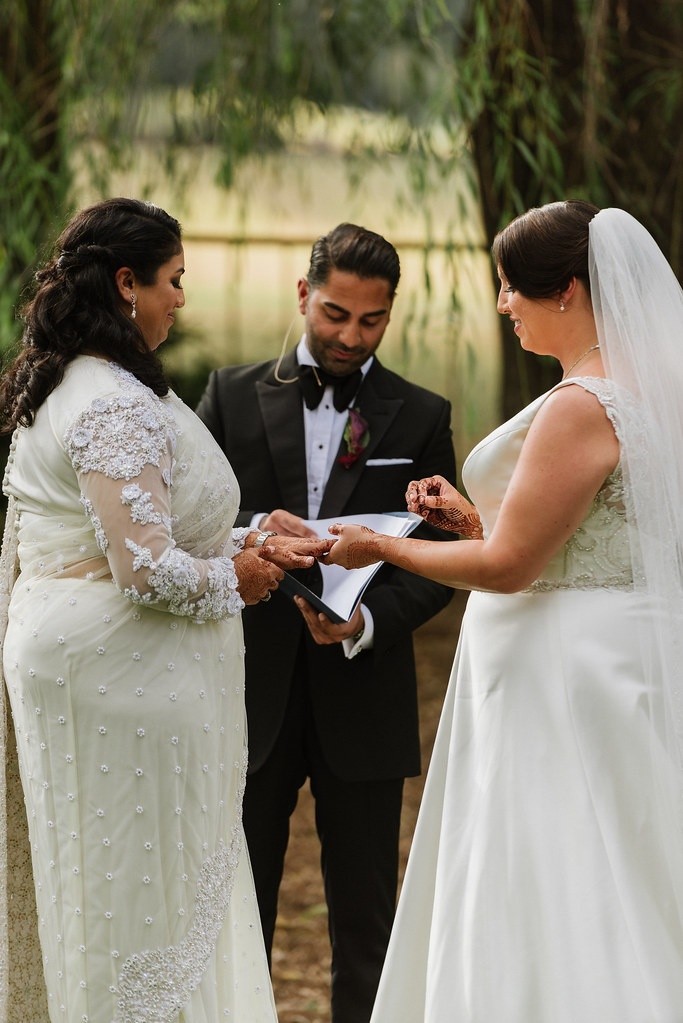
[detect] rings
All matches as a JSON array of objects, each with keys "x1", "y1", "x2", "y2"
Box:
[
  {"x1": 261, "y1": 591, "x2": 271, "y2": 602},
  {"x1": 416, "y1": 494, "x2": 425, "y2": 505}
]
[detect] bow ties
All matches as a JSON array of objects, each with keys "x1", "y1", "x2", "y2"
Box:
[{"x1": 297, "y1": 364, "x2": 363, "y2": 412}]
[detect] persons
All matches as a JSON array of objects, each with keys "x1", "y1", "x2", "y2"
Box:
[
  {"x1": 323, "y1": 202, "x2": 683, "y2": 1023},
  {"x1": 2, "y1": 196, "x2": 284, "y2": 1022},
  {"x1": 195, "y1": 223, "x2": 460, "y2": 1023}
]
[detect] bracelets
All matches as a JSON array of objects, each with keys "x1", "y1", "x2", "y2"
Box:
[{"x1": 253, "y1": 530, "x2": 277, "y2": 548}]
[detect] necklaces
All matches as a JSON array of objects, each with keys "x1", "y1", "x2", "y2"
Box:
[{"x1": 561, "y1": 344, "x2": 601, "y2": 381}]
